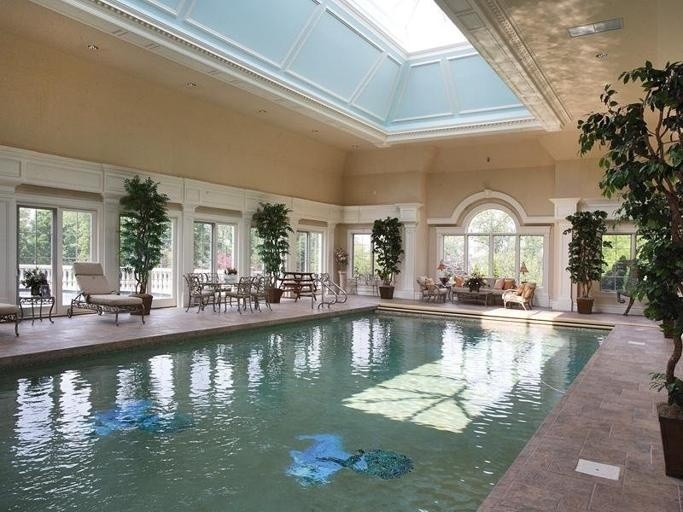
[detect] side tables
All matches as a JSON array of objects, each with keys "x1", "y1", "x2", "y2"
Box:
[{"x1": 19, "y1": 296, "x2": 55, "y2": 325}]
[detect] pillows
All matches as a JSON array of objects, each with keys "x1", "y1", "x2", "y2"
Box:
[
  {"x1": 425, "y1": 278, "x2": 434, "y2": 289},
  {"x1": 456, "y1": 277, "x2": 464, "y2": 286},
  {"x1": 503, "y1": 279, "x2": 514, "y2": 290},
  {"x1": 493, "y1": 278, "x2": 504, "y2": 290}
]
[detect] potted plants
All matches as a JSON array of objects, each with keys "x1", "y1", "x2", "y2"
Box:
[
  {"x1": 252, "y1": 202, "x2": 293, "y2": 303},
  {"x1": 562, "y1": 210, "x2": 611, "y2": 314},
  {"x1": 115, "y1": 175, "x2": 169, "y2": 316},
  {"x1": 371, "y1": 217, "x2": 404, "y2": 299},
  {"x1": 20, "y1": 268, "x2": 47, "y2": 294},
  {"x1": 578, "y1": 60, "x2": 683, "y2": 480},
  {"x1": 463, "y1": 273, "x2": 486, "y2": 292}
]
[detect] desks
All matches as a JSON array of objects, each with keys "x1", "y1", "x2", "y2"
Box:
[{"x1": 453, "y1": 290, "x2": 491, "y2": 308}]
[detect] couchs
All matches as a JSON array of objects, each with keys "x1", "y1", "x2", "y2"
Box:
[{"x1": 451, "y1": 276, "x2": 516, "y2": 295}]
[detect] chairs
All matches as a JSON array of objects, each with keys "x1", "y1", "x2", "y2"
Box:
[
  {"x1": 183, "y1": 273, "x2": 272, "y2": 317},
  {"x1": 417, "y1": 275, "x2": 449, "y2": 304},
  {"x1": 0, "y1": 303, "x2": 19, "y2": 337},
  {"x1": 67, "y1": 262, "x2": 146, "y2": 326},
  {"x1": 502, "y1": 281, "x2": 535, "y2": 310}
]
